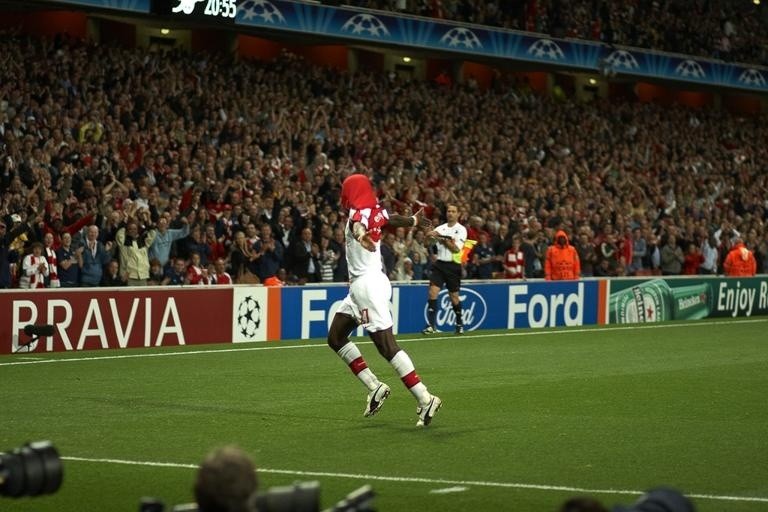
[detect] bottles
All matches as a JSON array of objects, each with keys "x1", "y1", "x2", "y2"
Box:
[{"x1": 609, "y1": 278, "x2": 714, "y2": 323}]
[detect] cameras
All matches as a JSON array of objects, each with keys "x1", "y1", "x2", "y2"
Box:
[{"x1": 0, "y1": 438, "x2": 62, "y2": 497}]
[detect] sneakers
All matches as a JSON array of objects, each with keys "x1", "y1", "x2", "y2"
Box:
[
  {"x1": 454, "y1": 325, "x2": 465, "y2": 335},
  {"x1": 414, "y1": 395, "x2": 443, "y2": 426},
  {"x1": 362, "y1": 381, "x2": 391, "y2": 419},
  {"x1": 421, "y1": 325, "x2": 439, "y2": 334}
]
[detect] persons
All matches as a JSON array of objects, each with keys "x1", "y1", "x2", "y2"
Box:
[
  {"x1": 422, "y1": 204, "x2": 467, "y2": 336},
  {"x1": 195, "y1": 443, "x2": 257, "y2": 509},
  {"x1": 0, "y1": 0, "x2": 766, "y2": 282},
  {"x1": 328, "y1": 174, "x2": 442, "y2": 428}
]
